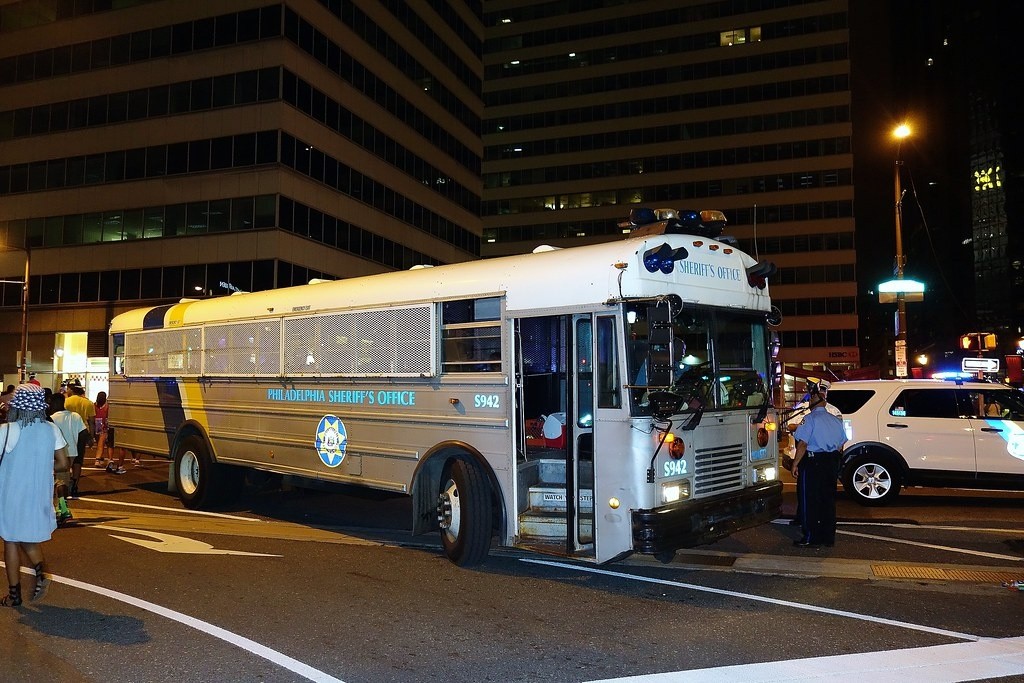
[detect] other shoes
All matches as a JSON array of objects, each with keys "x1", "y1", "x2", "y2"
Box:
[
  {"x1": 116, "y1": 467, "x2": 126, "y2": 474},
  {"x1": 55, "y1": 511, "x2": 63, "y2": 526},
  {"x1": 135, "y1": 462, "x2": 141, "y2": 466},
  {"x1": 106, "y1": 462, "x2": 114, "y2": 472},
  {"x1": 95, "y1": 460, "x2": 102, "y2": 468},
  {"x1": 131, "y1": 459, "x2": 136, "y2": 464},
  {"x1": 61, "y1": 509, "x2": 73, "y2": 526},
  {"x1": 793, "y1": 539, "x2": 834, "y2": 547},
  {"x1": 790, "y1": 520, "x2": 802, "y2": 528},
  {"x1": 66, "y1": 484, "x2": 78, "y2": 499}
]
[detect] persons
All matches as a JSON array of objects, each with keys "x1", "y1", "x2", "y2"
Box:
[
  {"x1": 786, "y1": 375, "x2": 848, "y2": 548},
  {"x1": 0, "y1": 371, "x2": 146, "y2": 527},
  {"x1": 634, "y1": 337, "x2": 686, "y2": 405},
  {"x1": 0, "y1": 383, "x2": 71, "y2": 608}
]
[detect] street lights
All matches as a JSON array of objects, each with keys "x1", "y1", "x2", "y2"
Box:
[{"x1": 893, "y1": 124, "x2": 913, "y2": 376}]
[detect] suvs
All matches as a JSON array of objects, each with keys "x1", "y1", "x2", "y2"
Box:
[{"x1": 783, "y1": 379, "x2": 1024, "y2": 508}]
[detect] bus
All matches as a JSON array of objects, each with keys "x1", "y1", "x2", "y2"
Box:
[{"x1": 107, "y1": 204, "x2": 793, "y2": 571}]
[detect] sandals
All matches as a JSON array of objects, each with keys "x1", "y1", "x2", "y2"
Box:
[
  {"x1": 0, "y1": 582, "x2": 23, "y2": 607},
  {"x1": 33, "y1": 561, "x2": 51, "y2": 603}
]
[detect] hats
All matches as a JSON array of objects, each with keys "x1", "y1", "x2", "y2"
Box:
[
  {"x1": 8, "y1": 383, "x2": 49, "y2": 411},
  {"x1": 67, "y1": 379, "x2": 84, "y2": 395},
  {"x1": 806, "y1": 376, "x2": 831, "y2": 392}
]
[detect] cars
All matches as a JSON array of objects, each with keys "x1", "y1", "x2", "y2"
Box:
[{"x1": 778, "y1": 370, "x2": 829, "y2": 440}]
